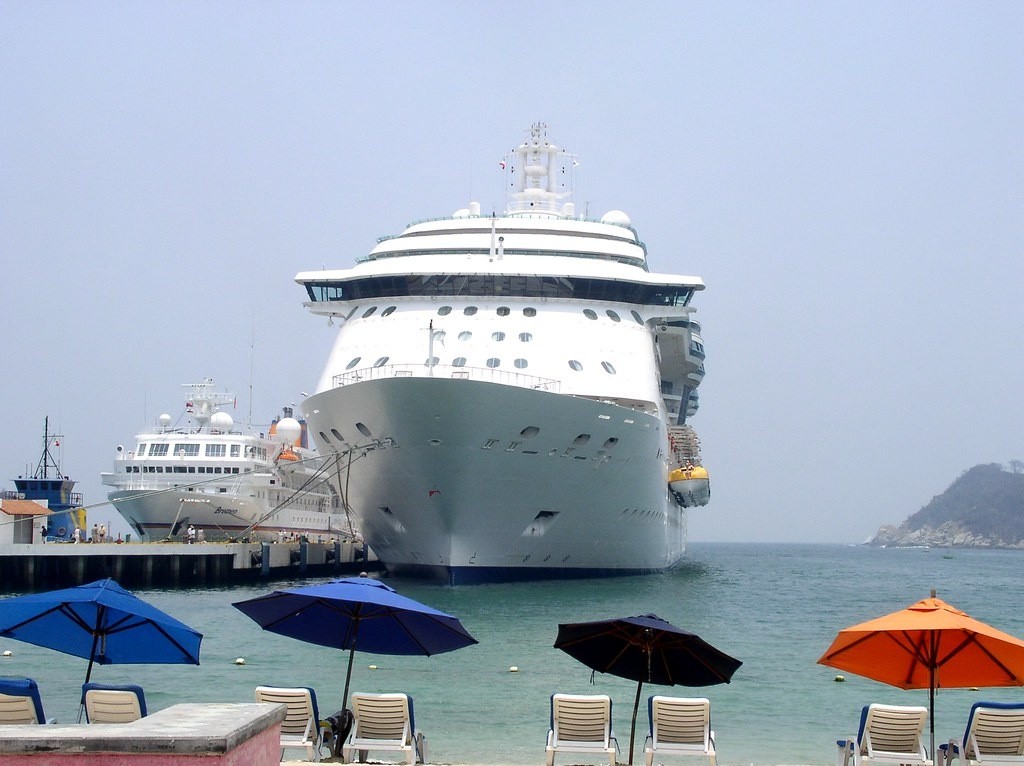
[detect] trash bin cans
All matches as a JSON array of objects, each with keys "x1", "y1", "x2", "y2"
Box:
[{"x1": 183, "y1": 535, "x2": 189, "y2": 544}]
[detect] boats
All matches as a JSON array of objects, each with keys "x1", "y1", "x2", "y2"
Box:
[
  {"x1": 8, "y1": 416, "x2": 88, "y2": 543},
  {"x1": 669, "y1": 466, "x2": 710, "y2": 509},
  {"x1": 275, "y1": 450, "x2": 301, "y2": 474}
]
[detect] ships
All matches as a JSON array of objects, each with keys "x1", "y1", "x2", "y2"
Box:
[
  {"x1": 99, "y1": 373, "x2": 364, "y2": 547},
  {"x1": 297, "y1": 116, "x2": 711, "y2": 587}
]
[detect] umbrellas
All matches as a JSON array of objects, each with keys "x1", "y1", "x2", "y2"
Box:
[
  {"x1": 232, "y1": 572, "x2": 479, "y2": 759},
  {"x1": 817, "y1": 588, "x2": 1024, "y2": 766},
  {"x1": 553, "y1": 613, "x2": 744, "y2": 766},
  {"x1": 0, "y1": 577, "x2": 205, "y2": 724}
]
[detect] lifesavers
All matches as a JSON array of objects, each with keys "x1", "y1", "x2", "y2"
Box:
[{"x1": 58, "y1": 527, "x2": 66, "y2": 536}]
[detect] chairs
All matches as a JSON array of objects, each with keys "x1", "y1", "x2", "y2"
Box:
[
  {"x1": 544, "y1": 694, "x2": 619, "y2": 766},
  {"x1": 937, "y1": 702, "x2": 1024, "y2": 766},
  {"x1": 82, "y1": 682, "x2": 148, "y2": 724},
  {"x1": 644, "y1": 696, "x2": 717, "y2": 766},
  {"x1": 0, "y1": 675, "x2": 58, "y2": 725},
  {"x1": 836, "y1": 702, "x2": 934, "y2": 766},
  {"x1": 254, "y1": 685, "x2": 338, "y2": 763},
  {"x1": 342, "y1": 691, "x2": 428, "y2": 765}
]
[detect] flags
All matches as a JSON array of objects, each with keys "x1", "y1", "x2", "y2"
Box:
[
  {"x1": 500, "y1": 159, "x2": 505, "y2": 169},
  {"x1": 186, "y1": 403, "x2": 193, "y2": 407}
]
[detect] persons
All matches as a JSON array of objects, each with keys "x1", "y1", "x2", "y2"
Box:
[
  {"x1": 322, "y1": 709, "x2": 355, "y2": 759},
  {"x1": 98, "y1": 524, "x2": 106, "y2": 543},
  {"x1": 198, "y1": 528, "x2": 204, "y2": 544},
  {"x1": 40, "y1": 526, "x2": 46, "y2": 544},
  {"x1": 90, "y1": 524, "x2": 98, "y2": 544},
  {"x1": 291, "y1": 529, "x2": 308, "y2": 541},
  {"x1": 668, "y1": 434, "x2": 694, "y2": 470},
  {"x1": 74, "y1": 526, "x2": 80, "y2": 544},
  {"x1": 188, "y1": 525, "x2": 195, "y2": 539},
  {"x1": 279, "y1": 529, "x2": 286, "y2": 543}
]
